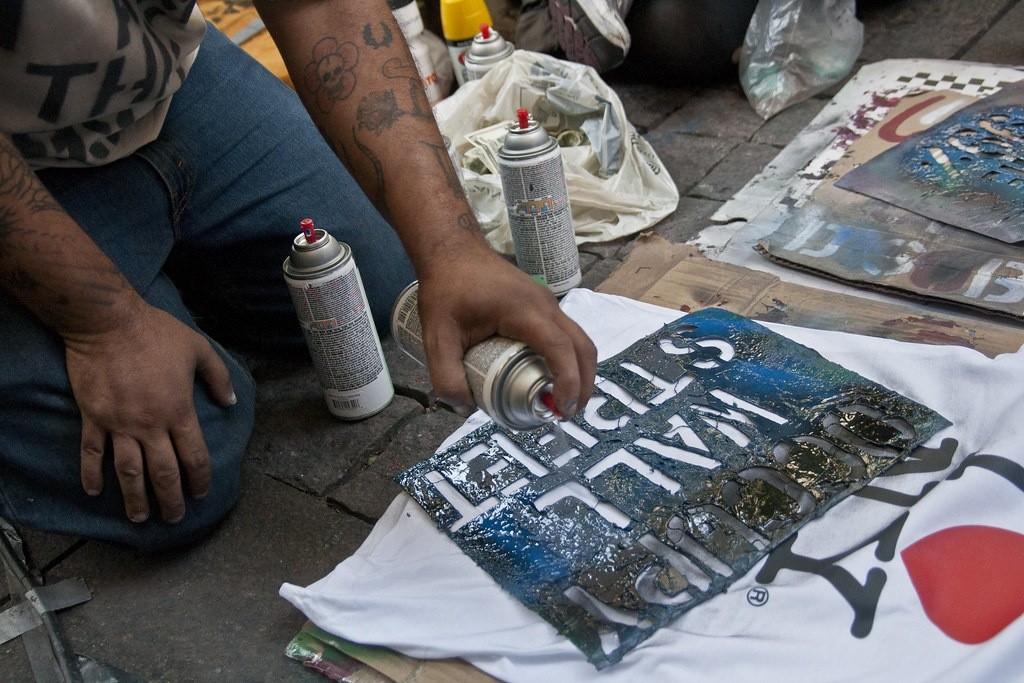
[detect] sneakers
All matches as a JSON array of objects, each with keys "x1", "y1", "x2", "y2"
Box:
[{"x1": 547, "y1": 1, "x2": 633, "y2": 74}]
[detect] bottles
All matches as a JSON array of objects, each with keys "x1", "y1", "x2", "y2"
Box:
[
  {"x1": 388, "y1": 277, "x2": 566, "y2": 429},
  {"x1": 281, "y1": 218, "x2": 396, "y2": 424},
  {"x1": 389, "y1": 1, "x2": 453, "y2": 112},
  {"x1": 465, "y1": 24, "x2": 514, "y2": 82},
  {"x1": 496, "y1": 108, "x2": 583, "y2": 300},
  {"x1": 438, "y1": 0, "x2": 494, "y2": 86}
]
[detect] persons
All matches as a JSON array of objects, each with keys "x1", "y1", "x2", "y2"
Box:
[{"x1": 0, "y1": 0, "x2": 601, "y2": 561}]
